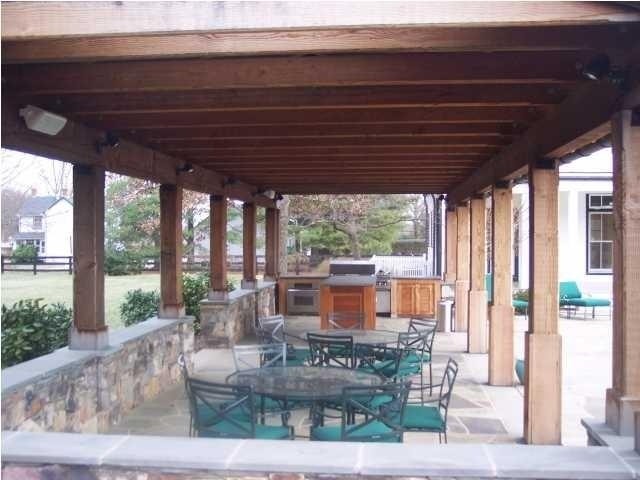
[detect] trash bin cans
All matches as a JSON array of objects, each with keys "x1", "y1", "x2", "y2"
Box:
[{"x1": 437, "y1": 300, "x2": 452, "y2": 332}]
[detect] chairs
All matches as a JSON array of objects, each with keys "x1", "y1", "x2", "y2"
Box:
[
  {"x1": 559, "y1": 281, "x2": 611, "y2": 320},
  {"x1": 486, "y1": 273, "x2": 528, "y2": 319},
  {"x1": 177, "y1": 314, "x2": 459, "y2": 441}
]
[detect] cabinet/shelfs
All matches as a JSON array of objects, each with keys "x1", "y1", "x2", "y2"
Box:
[
  {"x1": 390, "y1": 278, "x2": 441, "y2": 319},
  {"x1": 321, "y1": 285, "x2": 376, "y2": 330}
]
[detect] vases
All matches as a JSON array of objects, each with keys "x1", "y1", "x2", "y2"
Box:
[{"x1": 515, "y1": 358, "x2": 526, "y2": 385}]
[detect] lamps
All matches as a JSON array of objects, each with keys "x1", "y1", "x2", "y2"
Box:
[
  {"x1": 221, "y1": 176, "x2": 235, "y2": 189},
  {"x1": 252, "y1": 186, "x2": 283, "y2": 202},
  {"x1": 439, "y1": 195, "x2": 449, "y2": 202},
  {"x1": 96, "y1": 131, "x2": 120, "y2": 154},
  {"x1": 583, "y1": 53, "x2": 632, "y2": 89},
  {"x1": 176, "y1": 159, "x2": 194, "y2": 177},
  {"x1": 17, "y1": 104, "x2": 68, "y2": 137}
]
